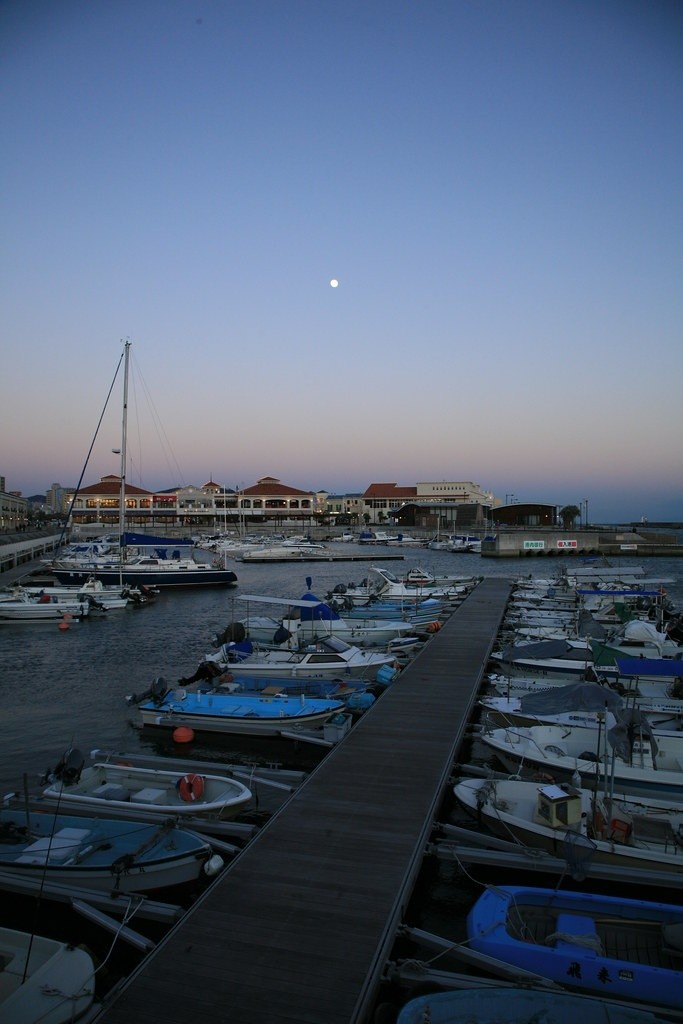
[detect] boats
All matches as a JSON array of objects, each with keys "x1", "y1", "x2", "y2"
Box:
[
  {"x1": 0, "y1": 337, "x2": 683, "y2": 699},
  {"x1": 39, "y1": 745, "x2": 253, "y2": 821},
  {"x1": 0, "y1": 793, "x2": 215, "y2": 893},
  {"x1": 125, "y1": 675, "x2": 347, "y2": 737},
  {"x1": 464, "y1": 884, "x2": 683, "y2": 1011},
  {"x1": 0, "y1": 925, "x2": 97, "y2": 1024},
  {"x1": 476, "y1": 682, "x2": 683, "y2": 739},
  {"x1": 453, "y1": 778, "x2": 683, "y2": 869},
  {"x1": 394, "y1": 987, "x2": 675, "y2": 1024},
  {"x1": 480, "y1": 725, "x2": 683, "y2": 794}
]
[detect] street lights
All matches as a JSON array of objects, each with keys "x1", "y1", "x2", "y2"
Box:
[
  {"x1": 585, "y1": 500, "x2": 588, "y2": 525},
  {"x1": 579, "y1": 503, "x2": 582, "y2": 527},
  {"x1": 510, "y1": 498, "x2": 518, "y2": 504},
  {"x1": 505, "y1": 493, "x2": 515, "y2": 505}
]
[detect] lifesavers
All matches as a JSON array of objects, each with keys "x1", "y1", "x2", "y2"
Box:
[{"x1": 179, "y1": 774, "x2": 203, "y2": 802}]
